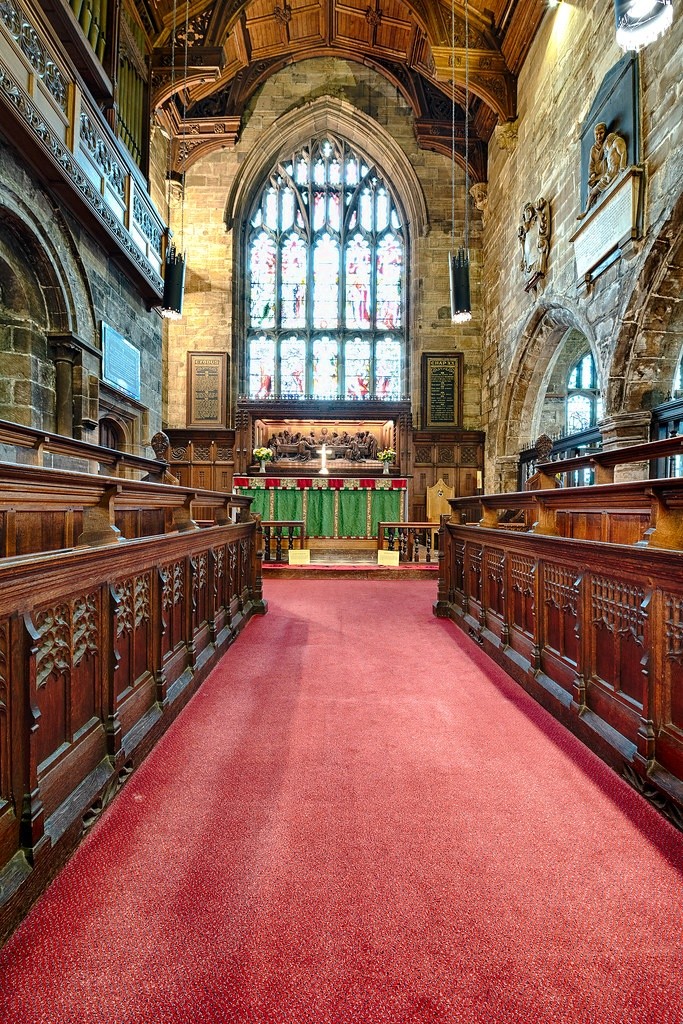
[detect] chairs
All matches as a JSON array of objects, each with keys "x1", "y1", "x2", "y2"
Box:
[{"x1": 425, "y1": 478, "x2": 455, "y2": 552}]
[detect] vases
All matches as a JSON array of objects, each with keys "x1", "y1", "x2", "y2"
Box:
[
  {"x1": 258, "y1": 459, "x2": 265, "y2": 472},
  {"x1": 382, "y1": 461, "x2": 389, "y2": 474}
]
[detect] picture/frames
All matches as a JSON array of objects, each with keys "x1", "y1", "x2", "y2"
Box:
[
  {"x1": 421, "y1": 351, "x2": 463, "y2": 429},
  {"x1": 186, "y1": 350, "x2": 226, "y2": 428}
]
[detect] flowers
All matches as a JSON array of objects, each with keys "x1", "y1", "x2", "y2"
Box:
[
  {"x1": 253, "y1": 446, "x2": 272, "y2": 460},
  {"x1": 376, "y1": 446, "x2": 396, "y2": 463}
]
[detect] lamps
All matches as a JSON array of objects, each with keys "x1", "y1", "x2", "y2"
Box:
[
  {"x1": 614, "y1": 0, "x2": 674, "y2": 52},
  {"x1": 162, "y1": 0, "x2": 185, "y2": 319},
  {"x1": 449, "y1": 0, "x2": 472, "y2": 324}
]
